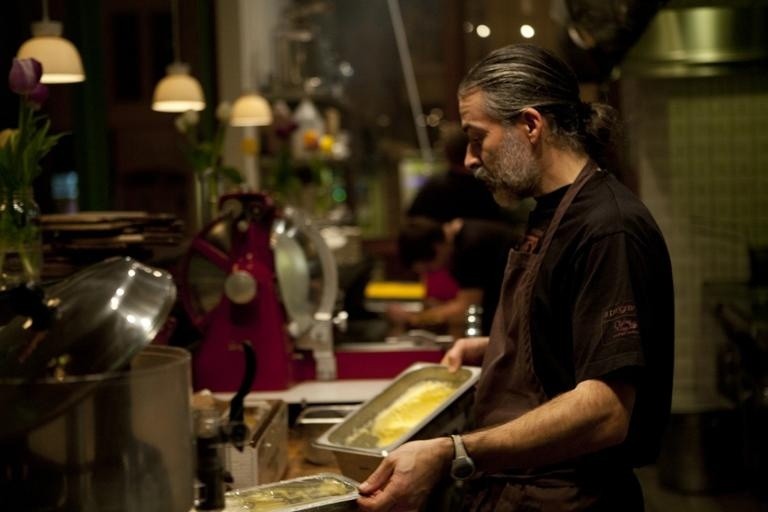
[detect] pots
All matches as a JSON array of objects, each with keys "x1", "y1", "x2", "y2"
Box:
[{"x1": 1, "y1": 250, "x2": 200, "y2": 512}]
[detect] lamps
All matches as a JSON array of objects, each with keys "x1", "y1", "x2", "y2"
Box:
[{"x1": 13, "y1": 2, "x2": 279, "y2": 130}]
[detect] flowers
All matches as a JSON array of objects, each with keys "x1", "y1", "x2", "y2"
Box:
[{"x1": 1, "y1": 53, "x2": 70, "y2": 287}]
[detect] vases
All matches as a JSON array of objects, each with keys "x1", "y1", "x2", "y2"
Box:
[{"x1": 2, "y1": 187, "x2": 44, "y2": 286}]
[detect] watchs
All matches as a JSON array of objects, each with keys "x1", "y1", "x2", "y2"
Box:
[{"x1": 447, "y1": 432, "x2": 476, "y2": 485}]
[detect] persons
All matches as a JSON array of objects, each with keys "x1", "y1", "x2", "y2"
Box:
[
  {"x1": 398, "y1": 125, "x2": 527, "y2": 227},
  {"x1": 353, "y1": 44, "x2": 684, "y2": 509},
  {"x1": 383, "y1": 216, "x2": 502, "y2": 335}
]
